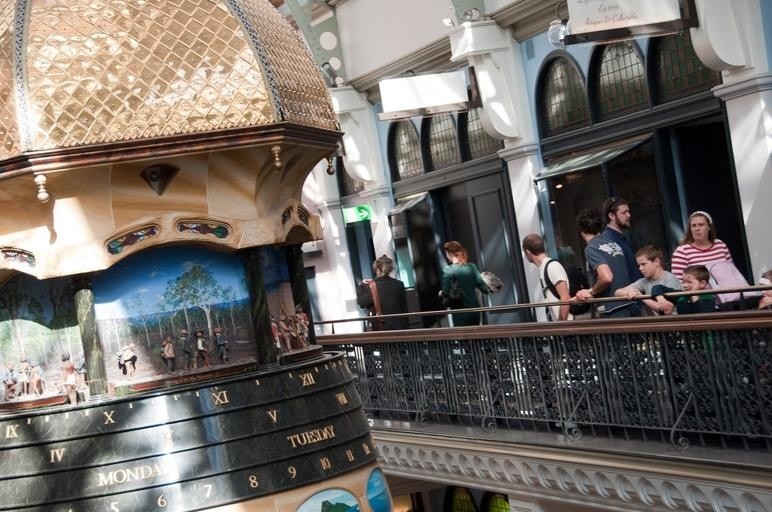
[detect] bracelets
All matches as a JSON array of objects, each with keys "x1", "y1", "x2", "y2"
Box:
[{"x1": 589, "y1": 287, "x2": 595, "y2": 297}]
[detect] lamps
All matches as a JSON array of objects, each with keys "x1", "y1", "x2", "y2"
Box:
[
  {"x1": 441, "y1": 17, "x2": 455, "y2": 31},
  {"x1": 543, "y1": 1, "x2": 571, "y2": 50}
]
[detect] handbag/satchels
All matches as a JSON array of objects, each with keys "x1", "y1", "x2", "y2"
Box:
[
  {"x1": 440, "y1": 265, "x2": 465, "y2": 315},
  {"x1": 365, "y1": 313, "x2": 385, "y2": 331}
]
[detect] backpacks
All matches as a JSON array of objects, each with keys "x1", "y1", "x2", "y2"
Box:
[{"x1": 543, "y1": 258, "x2": 592, "y2": 316}]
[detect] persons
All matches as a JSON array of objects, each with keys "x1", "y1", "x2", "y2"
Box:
[
  {"x1": 437, "y1": 240, "x2": 490, "y2": 354},
  {"x1": 553, "y1": 227, "x2": 585, "y2": 284},
  {"x1": 523, "y1": 233, "x2": 575, "y2": 359},
  {"x1": 651, "y1": 265, "x2": 722, "y2": 361},
  {"x1": 614, "y1": 244, "x2": 682, "y2": 317},
  {"x1": 601, "y1": 197, "x2": 650, "y2": 317},
  {"x1": 757, "y1": 269, "x2": 772, "y2": 309},
  {"x1": 574, "y1": 208, "x2": 636, "y2": 317},
  {"x1": 357, "y1": 255, "x2": 410, "y2": 376},
  {"x1": 670, "y1": 210, "x2": 761, "y2": 303}
]
